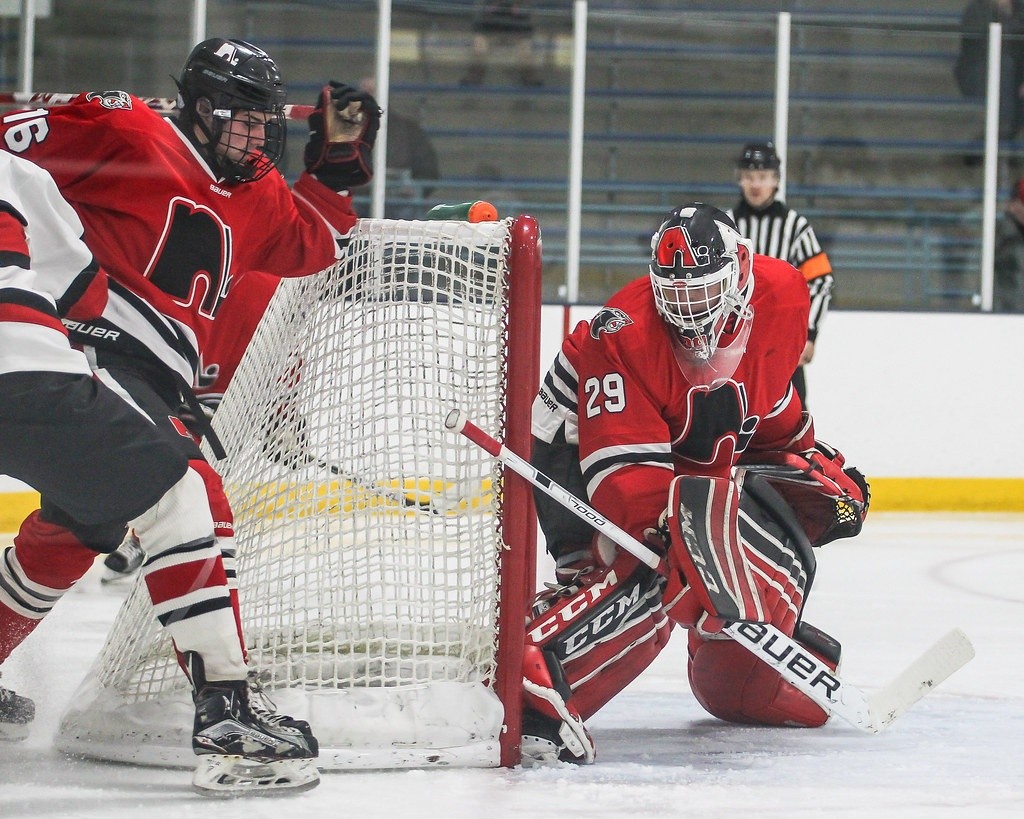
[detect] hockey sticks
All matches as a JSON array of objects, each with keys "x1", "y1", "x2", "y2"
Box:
[
  {"x1": 0, "y1": 90, "x2": 366, "y2": 125},
  {"x1": 180, "y1": 396, "x2": 480, "y2": 517},
  {"x1": 439, "y1": 405, "x2": 979, "y2": 737}
]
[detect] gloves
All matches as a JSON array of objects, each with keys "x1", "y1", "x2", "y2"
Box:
[{"x1": 304, "y1": 82, "x2": 384, "y2": 189}]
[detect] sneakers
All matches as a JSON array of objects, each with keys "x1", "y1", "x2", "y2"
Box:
[
  {"x1": 100, "y1": 529, "x2": 145, "y2": 585},
  {"x1": 0, "y1": 686, "x2": 36, "y2": 741},
  {"x1": 181, "y1": 650, "x2": 320, "y2": 791}
]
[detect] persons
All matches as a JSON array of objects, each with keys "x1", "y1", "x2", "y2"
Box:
[
  {"x1": 1, "y1": 35, "x2": 381, "y2": 799},
  {"x1": 346, "y1": 79, "x2": 441, "y2": 220},
  {"x1": 955, "y1": 1, "x2": 1024, "y2": 140},
  {"x1": 992, "y1": 179, "x2": 1023, "y2": 314},
  {"x1": 722, "y1": 141, "x2": 834, "y2": 410},
  {"x1": 484, "y1": 202, "x2": 870, "y2": 763},
  {"x1": 455, "y1": 0, "x2": 547, "y2": 89}
]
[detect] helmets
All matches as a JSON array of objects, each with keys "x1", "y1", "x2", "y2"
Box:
[
  {"x1": 737, "y1": 142, "x2": 781, "y2": 178},
  {"x1": 648, "y1": 201, "x2": 756, "y2": 390},
  {"x1": 168, "y1": 39, "x2": 286, "y2": 184}
]
